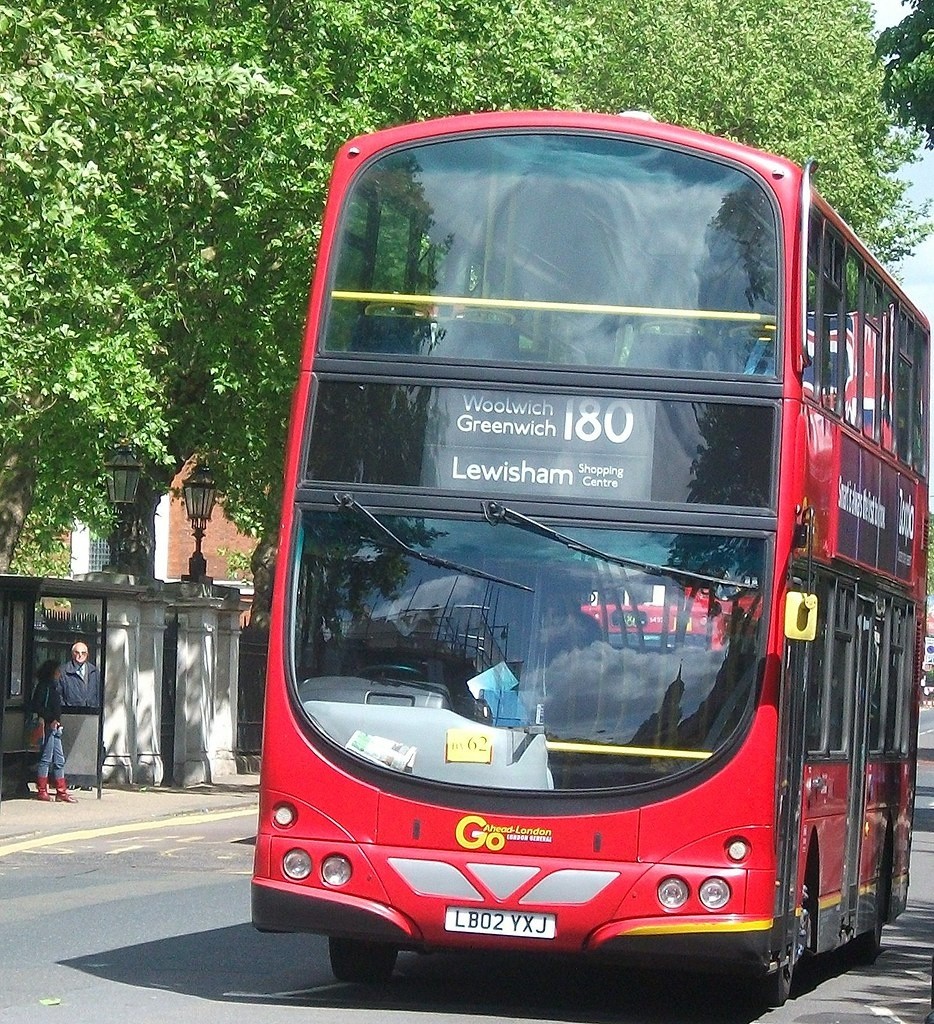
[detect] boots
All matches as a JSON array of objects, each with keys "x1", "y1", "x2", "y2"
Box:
[
  {"x1": 55, "y1": 778, "x2": 78, "y2": 802},
  {"x1": 37, "y1": 777, "x2": 53, "y2": 801}
]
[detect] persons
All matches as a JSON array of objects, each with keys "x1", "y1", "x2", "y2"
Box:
[
  {"x1": 30, "y1": 659, "x2": 79, "y2": 801},
  {"x1": 57, "y1": 642, "x2": 100, "y2": 791}
]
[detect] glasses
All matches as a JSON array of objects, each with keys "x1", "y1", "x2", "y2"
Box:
[{"x1": 73, "y1": 651, "x2": 87, "y2": 657}]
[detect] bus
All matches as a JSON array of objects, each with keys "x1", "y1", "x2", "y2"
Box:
[{"x1": 252, "y1": 113, "x2": 934, "y2": 1010}]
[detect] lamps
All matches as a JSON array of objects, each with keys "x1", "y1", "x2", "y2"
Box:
[
  {"x1": 181, "y1": 455, "x2": 219, "y2": 585},
  {"x1": 101, "y1": 435, "x2": 144, "y2": 576}
]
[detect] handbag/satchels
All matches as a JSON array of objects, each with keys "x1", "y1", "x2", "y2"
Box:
[{"x1": 23, "y1": 713, "x2": 45, "y2": 748}]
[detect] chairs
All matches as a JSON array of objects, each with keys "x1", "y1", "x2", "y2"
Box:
[{"x1": 349, "y1": 301, "x2": 785, "y2": 380}]
